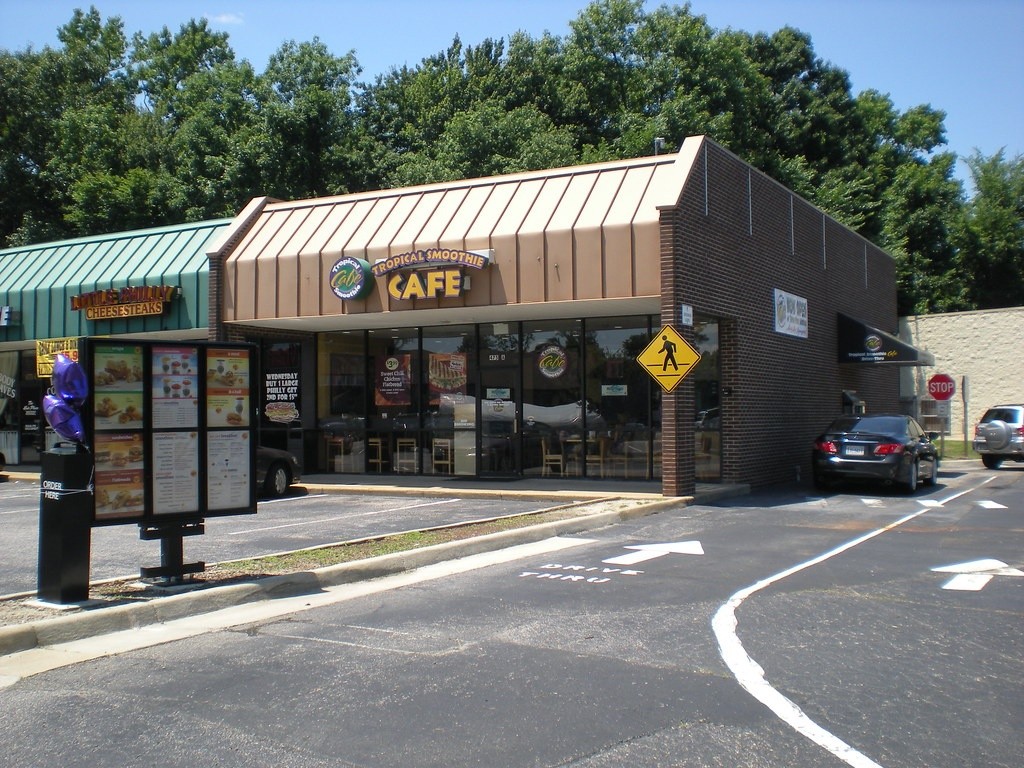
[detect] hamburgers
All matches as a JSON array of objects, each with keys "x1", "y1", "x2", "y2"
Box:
[
  {"x1": 264, "y1": 402, "x2": 300, "y2": 423},
  {"x1": 95, "y1": 354, "x2": 249, "y2": 509}
]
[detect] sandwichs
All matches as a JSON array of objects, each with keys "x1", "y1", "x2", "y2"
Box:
[{"x1": 430, "y1": 355, "x2": 466, "y2": 390}]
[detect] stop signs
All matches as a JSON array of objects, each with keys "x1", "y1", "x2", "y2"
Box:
[{"x1": 928, "y1": 373, "x2": 956, "y2": 401}]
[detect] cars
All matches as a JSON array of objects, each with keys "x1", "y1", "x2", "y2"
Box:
[
  {"x1": 254, "y1": 444, "x2": 303, "y2": 497},
  {"x1": 809, "y1": 412, "x2": 940, "y2": 495}
]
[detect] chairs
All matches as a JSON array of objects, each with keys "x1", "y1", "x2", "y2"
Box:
[
  {"x1": 323, "y1": 434, "x2": 455, "y2": 478},
  {"x1": 539, "y1": 425, "x2": 721, "y2": 479}
]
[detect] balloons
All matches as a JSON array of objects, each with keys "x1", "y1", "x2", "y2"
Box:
[
  {"x1": 51, "y1": 352, "x2": 89, "y2": 410},
  {"x1": 43, "y1": 395, "x2": 85, "y2": 445}
]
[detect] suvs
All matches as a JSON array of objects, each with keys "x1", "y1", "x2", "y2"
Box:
[{"x1": 971, "y1": 403, "x2": 1024, "y2": 472}]
